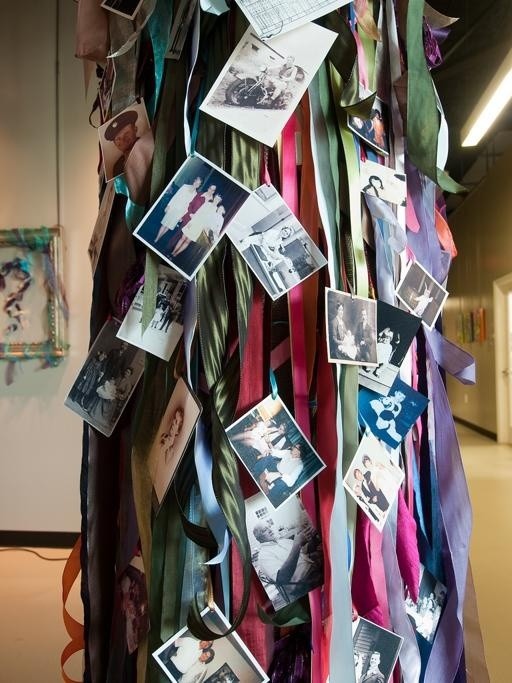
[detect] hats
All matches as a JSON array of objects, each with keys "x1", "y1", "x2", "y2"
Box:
[
  {"x1": 104, "y1": 109, "x2": 139, "y2": 143},
  {"x1": 382, "y1": 330, "x2": 394, "y2": 341}
]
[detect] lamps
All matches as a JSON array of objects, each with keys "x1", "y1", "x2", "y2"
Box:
[{"x1": 458, "y1": 44, "x2": 512, "y2": 149}]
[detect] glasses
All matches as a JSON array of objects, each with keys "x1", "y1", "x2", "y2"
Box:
[{"x1": 292, "y1": 444, "x2": 300, "y2": 451}]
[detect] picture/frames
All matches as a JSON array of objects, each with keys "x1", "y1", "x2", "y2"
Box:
[{"x1": 0, "y1": 226, "x2": 65, "y2": 360}]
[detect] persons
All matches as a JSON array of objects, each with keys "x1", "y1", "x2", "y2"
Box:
[
  {"x1": 73, "y1": 55, "x2": 442, "y2": 682},
  {"x1": 0, "y1": 257, "x2": 34, "y2": 343}
]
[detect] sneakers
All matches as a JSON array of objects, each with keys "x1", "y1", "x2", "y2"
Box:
[
  {"x1": 168, "y1": 253, "x2": 177, "y2": 262},
  {"x1": 263, "y1": 99, "x2": 273, "y2": 105}
]
